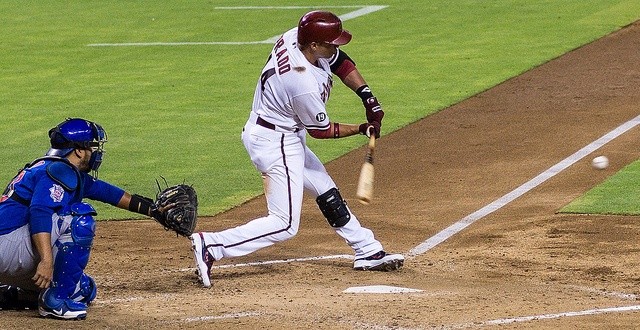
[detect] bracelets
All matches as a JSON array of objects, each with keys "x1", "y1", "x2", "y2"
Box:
[
  {"x1": 129, "y1": 193, "x2": 153, "y2": 216},
  {"x1": 355, "y1": 84, "x2": 374, "y2": 102}
]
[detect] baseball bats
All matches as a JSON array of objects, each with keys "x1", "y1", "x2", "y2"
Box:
[{"x1": 356, "y1": 131, "x2": 375, "y2": 206}]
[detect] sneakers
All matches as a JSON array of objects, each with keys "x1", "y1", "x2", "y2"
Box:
[
  {"x1": 38, "y1": 288, "x2": 87, "y2": 320},
  {"x1": 189, "y1": 229, "x2": 215, "y2": 288},
  {"x1": 354, "y1": 251, "x2": 404, "y2": 272}
]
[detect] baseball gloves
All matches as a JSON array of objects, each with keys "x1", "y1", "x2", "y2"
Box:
[{"x1": 150, "y1": 175, "x2": 199, "y2": 240}]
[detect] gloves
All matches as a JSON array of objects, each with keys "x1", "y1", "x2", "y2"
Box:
[
  {"x1": 363, "y1": 96, "x2": 384, "y2": 127},
  {"x1": 359, "y1": 121, "x2": 380, "y2": 140}
]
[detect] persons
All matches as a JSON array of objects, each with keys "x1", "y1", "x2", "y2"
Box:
[
  {"x1": 0, "y1": 117, "x2": 196, "y2": 319},
  {"x1": 189, "y1": 10, "x2": 406, "y2": 287}
]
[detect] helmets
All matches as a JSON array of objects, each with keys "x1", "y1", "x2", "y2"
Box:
[
  {"x1": 298, "y1": 11, "x2": 352, "y2": 46},
  {"x1": 44, "y1": 117, "x2": 108, "y2": 182}
]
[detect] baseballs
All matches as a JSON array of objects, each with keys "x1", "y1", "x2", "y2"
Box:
[{"x1": 592, "y1": 156, "x2": 609, "y2": 170}]
